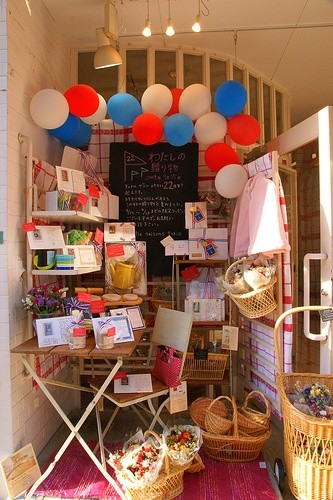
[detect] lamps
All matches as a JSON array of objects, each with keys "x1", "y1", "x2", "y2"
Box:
[{"x1": 94, "y1": 27, "x2": 122, "y2": 69}]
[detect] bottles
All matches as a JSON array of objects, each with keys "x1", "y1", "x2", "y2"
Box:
[
  {"x1": 96, "y1": 322, "x2": 115, "y2": 349},
  {"x1": 67, "y1": 310, "x2": 87, "y2": 349}
]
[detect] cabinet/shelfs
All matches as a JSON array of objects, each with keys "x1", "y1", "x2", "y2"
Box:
[
  {"x1": 19, "y1": 132, "x2": 108, "y2": 276},
  {"x1": 171, "y1": 251, "x2": 234, "y2": 399}
]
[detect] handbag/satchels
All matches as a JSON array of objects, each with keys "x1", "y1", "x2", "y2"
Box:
[{"x1": 151, "y1": 345, "x2": 183, "y2": 388}]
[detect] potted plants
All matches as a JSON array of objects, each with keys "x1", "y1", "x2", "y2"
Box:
[{"x1": 152, "y1": 281, "x2": 176, "y2": 311}]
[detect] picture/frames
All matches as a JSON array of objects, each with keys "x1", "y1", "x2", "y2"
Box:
[{"x1": 198, "y1": 171, "x2": 231, "y2": 224}]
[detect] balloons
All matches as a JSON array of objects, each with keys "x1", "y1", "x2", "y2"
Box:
[{"x1": 28, "y1": 81, "x2": 261, "y2": 197}]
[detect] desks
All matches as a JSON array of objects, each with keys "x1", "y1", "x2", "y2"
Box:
[{"x1": 11, "y1": 321, "x2": 150, "y2": 500}]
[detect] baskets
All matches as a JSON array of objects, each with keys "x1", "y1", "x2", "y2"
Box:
[
  {"x1": 177, "y1": 352, "x2": 230, "y2": 381},
  {"x1": 121, "y1": 430, "x2": 205, "y2": 500},
  {"x1": 274, "y1": 306, "x2": 333, "y2": 500},
  {"x1": 188, "y1": 389, "x2": 273, "y2": 463},
  {"x1": 223, "y1": 257, "x2": 278, "y2": 319}
]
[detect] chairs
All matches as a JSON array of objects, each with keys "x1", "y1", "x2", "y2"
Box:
[{"x1": 89, "y1": 304, "x2": 194, "y2": 470}]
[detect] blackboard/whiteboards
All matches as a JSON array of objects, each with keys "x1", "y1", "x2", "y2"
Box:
[{"x1": 108, "y1": 141, "x2": 200, "y2": 277}]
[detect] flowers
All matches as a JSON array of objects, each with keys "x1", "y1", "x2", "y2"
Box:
[{"x1": 22, "y1": 282, "x2": 66, "y2": 316}]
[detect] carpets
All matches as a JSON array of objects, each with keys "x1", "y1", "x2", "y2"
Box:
[{"x1": 29, "y1": 439, "x2": 280, "y2": 500}]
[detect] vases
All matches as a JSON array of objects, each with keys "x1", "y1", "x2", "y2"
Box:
[{"x1": 35, "y1": 312, "x2": 57, "y2": 318}]
